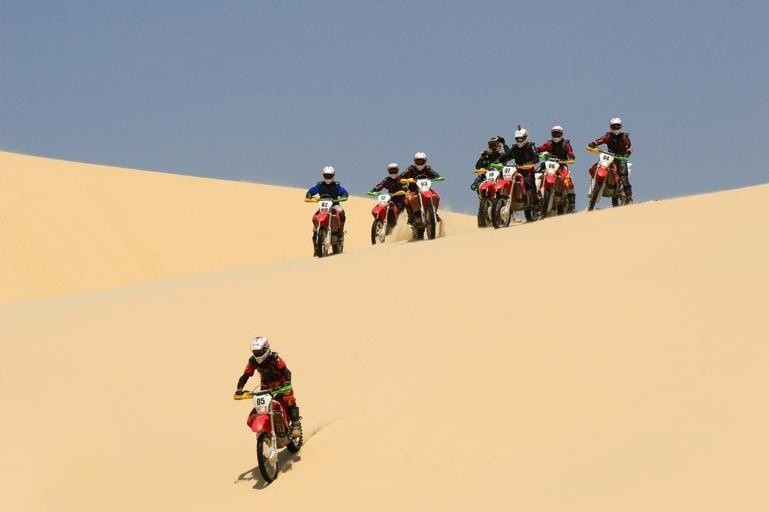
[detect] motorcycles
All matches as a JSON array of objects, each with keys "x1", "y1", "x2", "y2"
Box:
[
  {"x1": 232, "y1": 381, "x2": 304, "y2": 484},
  {"x1": 472, "y1": 147, "x2": 632, "y2": 229},
  {"x1": 305, "y1": 193, "x2": 350, "y2": 259},
  {"x1": 397, "y1": 175, "x2": 446, "y2": 242},
  {"x1": 366, "y1": 192, "x2": 406, "y2": 244}
]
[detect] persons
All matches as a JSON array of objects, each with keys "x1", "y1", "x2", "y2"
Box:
[
  {"x1": 305, "y1": 165, "x2": 349, "y2": 258},
  {"x1": 371, "y1": 162, "x2": 408, "y2": 235},
  {"x1": 535, "y1": 125, "x2": 578, "y2": 220},
  {"x1": 235, "y1": 335, "x2": 302, "y2": 440},
  {"x1": 474, "y1": 137, "x2": 506, "y2": 180},
  {"x1": 588, "y1": 117, "x2": 633, "y2": 206},
  {"x1": 398, "y1": 151, "x2": 441, "y2": 226},
  {"x1": 497, "y1": 124, "x2": 546, "y2": 221}
]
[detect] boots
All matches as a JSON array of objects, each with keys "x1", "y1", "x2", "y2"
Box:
[
  {"x1": 622, "y1": 184, "x2": 633, "y2": 206},
  {"x1": 566, "y1": 193, "x2": 575, "y2": 214}
]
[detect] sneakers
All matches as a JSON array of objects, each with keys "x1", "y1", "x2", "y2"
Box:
[
  {"x1": 313, "y1": 243, "x2": 320, "y2": 257},
  {"x1": 337, "y1": 229, "x2": 345, "y2": 241},
  {"x1": 290, "y1": 424, "x2": 302, "y2": 438}
]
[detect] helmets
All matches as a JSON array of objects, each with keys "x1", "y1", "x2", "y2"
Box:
[
  {"x1": 386, "y1": 162, "x2": 400, "y2": 180},
  {"x1": 608, "y1": 117, "x2": 623, "y2": 136},
  {"x1": 488, "y1": 137, "x2": 502, "y2": 153},
  {"x1": 513, "y1": 128, "x2": 528, "y2": 148},
  {"x1": 549, "y1": 125, "x2": 563, "y2": 143},
  {"x1": 413, "y1": 152, "x2": 427, "y2": 171},
  {"x1": 249, "y1": 336, "x2": 271, "y2": 364},
  {"x1": 321, "y1": 166, "x2": 335, "y2": 184}
]
